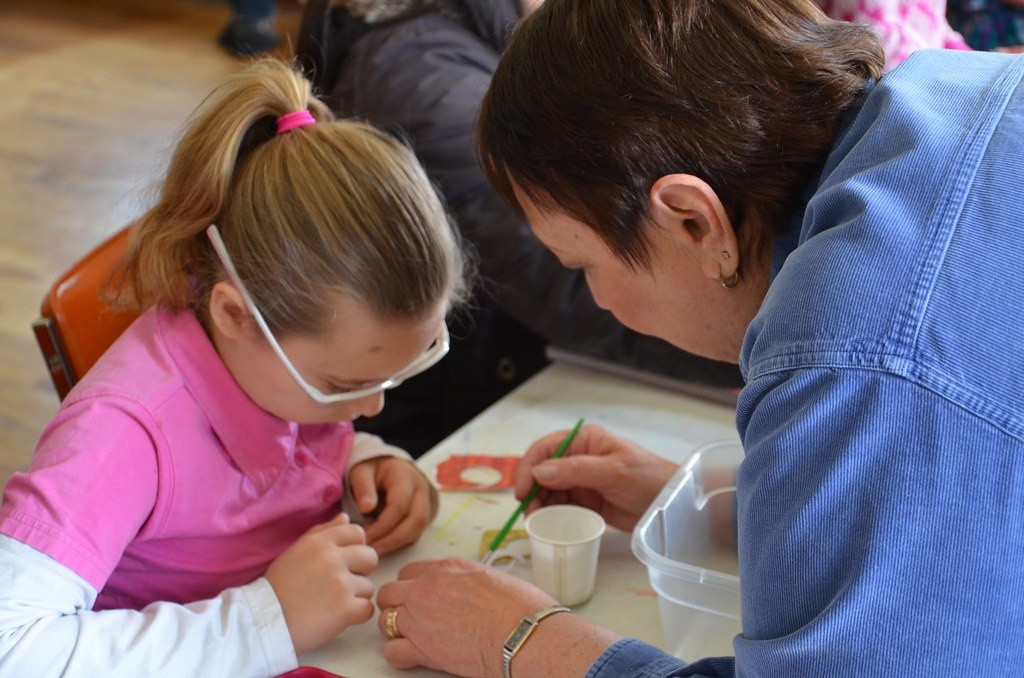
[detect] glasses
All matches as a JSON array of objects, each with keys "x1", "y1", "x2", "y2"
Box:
[{"x1": 206, "y1": 225, "x2": 450, "y2": 406}]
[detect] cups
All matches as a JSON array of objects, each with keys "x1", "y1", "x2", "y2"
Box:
[{"x1": 524, "y1": 504, "x2": 606, "y2": 605}]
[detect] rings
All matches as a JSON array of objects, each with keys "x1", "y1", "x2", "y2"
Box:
[{"x1": 386, "y1": 609, "x2": 401, "y2": 639}]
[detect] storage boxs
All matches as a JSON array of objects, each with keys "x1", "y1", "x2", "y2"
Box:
[{"x1": 630, "y1": 440, "x2": 745, "y2": 622}]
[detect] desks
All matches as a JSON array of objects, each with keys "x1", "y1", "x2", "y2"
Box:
[{"x1": 299, "y1": 358, "x2": 742, "y2": 677}]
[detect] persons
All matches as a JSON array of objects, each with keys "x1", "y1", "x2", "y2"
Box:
[
  {"x1": 814, "y1": 0, "x2": 1024, "y2": 74},
  {"x1": 325, "y1": 0, "x2": 745, "y2": 461},
  {"x1": 0, "y1": 52, "x2": 460, "y2": 678},
  {"x1": 376, "y1": 0, "x2": 1023, "y2": 678}
]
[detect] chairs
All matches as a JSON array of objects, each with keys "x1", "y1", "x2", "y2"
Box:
[{"x1": 31, "y1": 204, "x2": 153, "y2": 406}]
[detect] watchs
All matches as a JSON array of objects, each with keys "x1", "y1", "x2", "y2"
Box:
[{"x1": 502, "y1": 604, "x2": 572, "y2": 678}]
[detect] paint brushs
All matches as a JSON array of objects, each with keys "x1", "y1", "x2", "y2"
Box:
[{"x1": 471, "y1": 416, "x2": 587, "y2": 566}]
